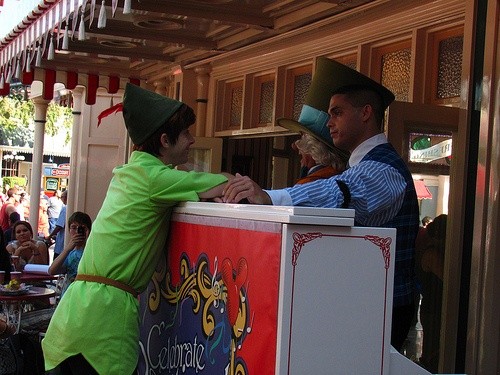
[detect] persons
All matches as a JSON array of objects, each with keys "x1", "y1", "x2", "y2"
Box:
[
  {"x1": 0, "y1": 183, "x2": 67, "y2": 261},
  {"x1": 6, "y1": 220, "x2": 48, "y2": 308},
  {"x1": 224, "y1": 74, "x2": 419, "y2": 350},
  {"x1": 276, "y1": 56, "x2": 395, "y2": 183},
  {"x1": 416, "y1": 215, "x2": 448, "y2": 374},
  {"x1": 420, "y1": 215, "x2": 433, "y2": 235},
  {"x1": 42, "y1": 81, "x2": 233, "y2": 375},
  {"x1": 48, "y1": 211, "x2": 92, "y2": 293}
]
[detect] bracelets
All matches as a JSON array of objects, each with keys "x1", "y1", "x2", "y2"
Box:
[{"x1": 34, "y1": 253, "x2": 40, "y2": 256}]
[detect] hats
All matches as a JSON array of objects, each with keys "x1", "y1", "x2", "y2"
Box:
[
  {"x1": 277, "y1": 56, "x2": 395, "y2": 159},
  {"x1": 123, "y1": 83, "x2": 183, "y2": 145}
]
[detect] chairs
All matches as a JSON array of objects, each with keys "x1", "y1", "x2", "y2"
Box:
[{"x1": 0, "y1": 272, "x2": 69, "y2": 375}]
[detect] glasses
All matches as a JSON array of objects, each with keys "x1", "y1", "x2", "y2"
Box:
[{"x1": 68, "y1": 226, "x2": 88, "y2": 232}]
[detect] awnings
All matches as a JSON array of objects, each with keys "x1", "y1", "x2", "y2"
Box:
[{"x1": 413, "y1": 180, "x2": 432, "y2": 199}]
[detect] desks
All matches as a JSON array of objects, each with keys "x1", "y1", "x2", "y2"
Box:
[{"x1": 0, "y1": 286, "x2": 56, "y2": 334}]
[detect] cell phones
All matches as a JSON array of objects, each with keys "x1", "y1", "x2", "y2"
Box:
[{"x1": 77, "y1": 225, "x2": 86, "y2": 239}]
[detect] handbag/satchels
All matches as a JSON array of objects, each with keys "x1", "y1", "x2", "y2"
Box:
[{"x1": 0, "y1": 338, "x2": 19, "y2": 375}]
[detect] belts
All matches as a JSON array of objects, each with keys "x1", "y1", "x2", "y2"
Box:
[{"x1": 75, "y1": 275, "x2": 137, "y2": 300}]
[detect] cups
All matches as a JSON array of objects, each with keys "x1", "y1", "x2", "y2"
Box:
[
  {"x1": 0, "y1": 271, "x2": 5, "y2": 285},
  {"x1": 10, "y1": 272, "x2": 22, "y2": 288},
  {"x1": 11, "y1": 256, "x2": 18, "y2": 266}
]
